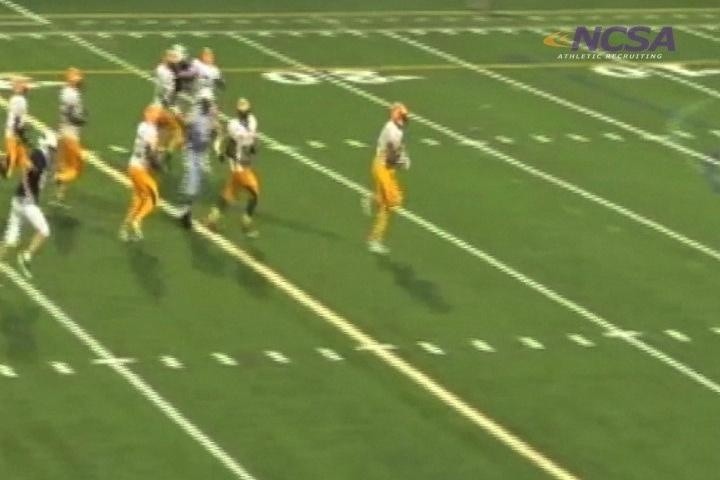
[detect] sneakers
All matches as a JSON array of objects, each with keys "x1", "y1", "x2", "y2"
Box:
[
  {"x1": 17, "y1": 252, "x2": 33, "y2": 278},
  {"x1": 176, "y1": 206, "x2": 260, "y2": 239},
  {"x1": 119, "y1": 221, "x2": 144, "y2": 242},
  {"x1": 367, "y1": 240, "x2": 389, "y2": 254}
]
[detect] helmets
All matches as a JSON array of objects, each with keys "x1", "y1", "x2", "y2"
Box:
[
  {"x1": 165, "y1": 44, "x2": 215, "y2": 65},
  {"x1": 38, "y1": 133, "x2": 58, "y2": 152},
  {"x1": 143, "y1": 88, "x2": 251, "y2": 125},
  {"x1": 65, "y1": 67, "x2": 83, "y2": 81},
  {"x1": 391, "y1": 103, "x2": 409, "y2": 126}
]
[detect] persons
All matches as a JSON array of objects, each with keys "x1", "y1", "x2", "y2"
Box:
[
  {"x1": 3, "y1": 77, "x2": 35, "y2": 179},
  {"x1": 119, "y1": 43, "x2": 261, "y2": 241},
  {"x1": 0, "y1": 131, "x2": 57, "y2": 278},
  {"x1": 47, "y1": 66, "x2": 88, "y2": 209},
  {"x1": 363, "y1": 103, "x2": 411, "y2": 255}
]
